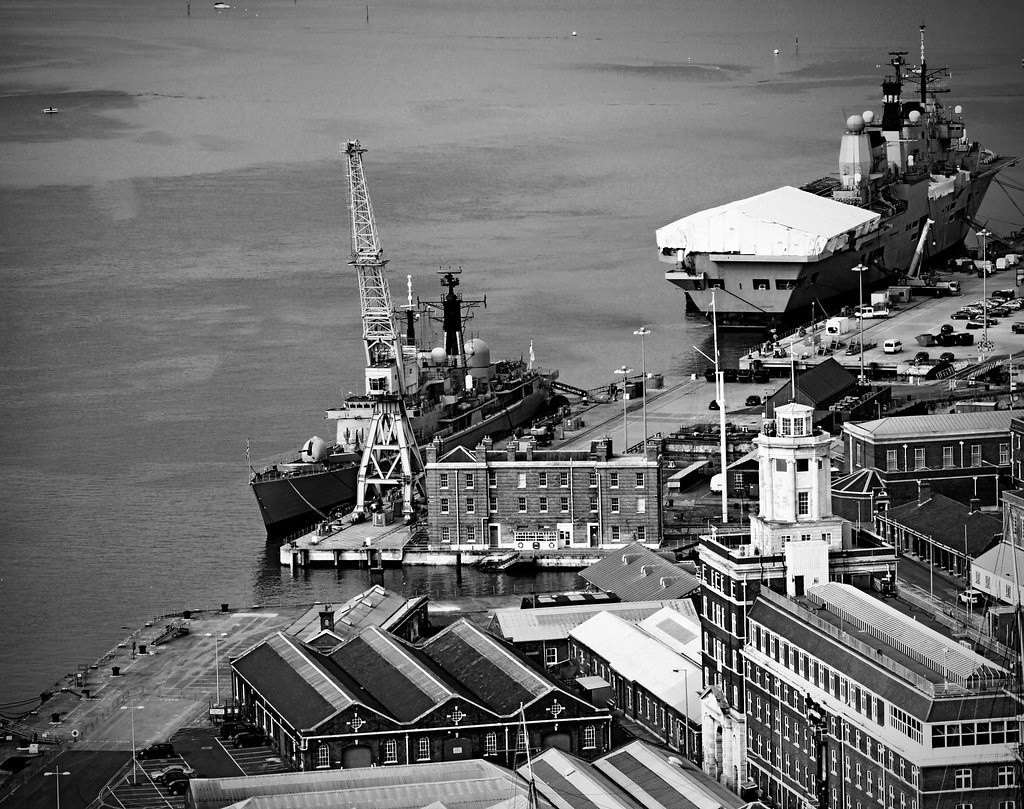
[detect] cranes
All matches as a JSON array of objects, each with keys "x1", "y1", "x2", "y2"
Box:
[{"x1": 341, "y1": 138, "x2": 427, "y2": 527}]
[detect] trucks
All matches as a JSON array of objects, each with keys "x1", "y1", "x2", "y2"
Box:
[
  {"x1": 996, "y1": 258, "x2": 1010, "y2": 270},
  {"x1": 983, "y1": 264, "x2": 996, "y2": 274},
  {"x1": 706, "y1": 360, "x2": 770, "y2": 384},
  {"x1": 854, "y1": 306, "x2": 889, "y2": 319},
  {"x1": 1006, "y1": 254, "x2": 1019, "y2": 267}
]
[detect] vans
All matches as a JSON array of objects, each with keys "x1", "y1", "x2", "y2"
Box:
[
  {"x1": 773, "y1": 347, "x2": 786, "y2": 358},
  {"x1": 138, "y1": 742, "x2": 174, "y2": 759},
  {"x1": 992, "y1": 289, "x2": 1014, "y2": 300},
  {"x1": 220, "y1": 724, "x2": 250, "y2": 740},
  {"x1": 883, "y1": 340, "x2": 902, "y2": 354},
  {"x1": 978, "y1": 269, "x2": 990, "y2": 278}
]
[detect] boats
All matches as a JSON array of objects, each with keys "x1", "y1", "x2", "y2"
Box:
[
  {"x1": 246, "y1": 264, "x2": 552, "y2": 532},
  {"x1": 655, "y1": 20, "x2": 1020, "y2": 327}
]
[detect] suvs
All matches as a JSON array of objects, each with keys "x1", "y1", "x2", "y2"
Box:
[
  {"x1": 234, "y1": 732, "x2": 268, "y2": 748},
  {"x1": 975, "y1": 315, "x2": 996, "y2": 323},
  {"x1": 162, "y1": 771, "x2": 194, "y2": 784}
]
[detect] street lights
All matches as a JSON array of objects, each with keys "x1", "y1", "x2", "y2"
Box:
[
  {"x1": 615, "y1": 365, "x2": 632, "y2": 453},
  {"x1": 976, "y1": 229, "x2": 992, "y2": 345},
  {"x1": 635, "y1": 326, "x2": 651, "y2": 453},
  {"x1": 851, "y1": 263, "x2": 868, "y2": 385}
]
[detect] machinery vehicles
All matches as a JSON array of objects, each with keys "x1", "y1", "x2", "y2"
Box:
[{"x1": 898, "y1": 217, "x2": 960, "y2": 296}]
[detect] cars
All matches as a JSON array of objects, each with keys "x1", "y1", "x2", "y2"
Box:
[
  {"x1": 167, "y1": 780, "x2": 191, "y2": 795},
  {"x1": 915, "y1": 352, "x2": 928, "y2": 360},
  {"x1": 961, "y1": 306, "x2": 983, "y2": 314},
  {"x1": 951, "y1": 311, "x2": 973, "y2": 320},
  {"x1": 941, "y1": 325, "x2": 953, "y2": 333},
  {"x1": 969, "y1": 296, "x2": 1024, "y2": 312},
  {"x1": 1012, "y1": 322, "x2": 1024, "y2": 333},
  {"x1": 989, "y1": 309, "x2": 1008, "y2": 317},
  {"x1": 152, "y1": 765, "x2": 195, "y2": 781},
  {"x1": 939, "y1": 352, "x2": 954, "y2": 362}
]
